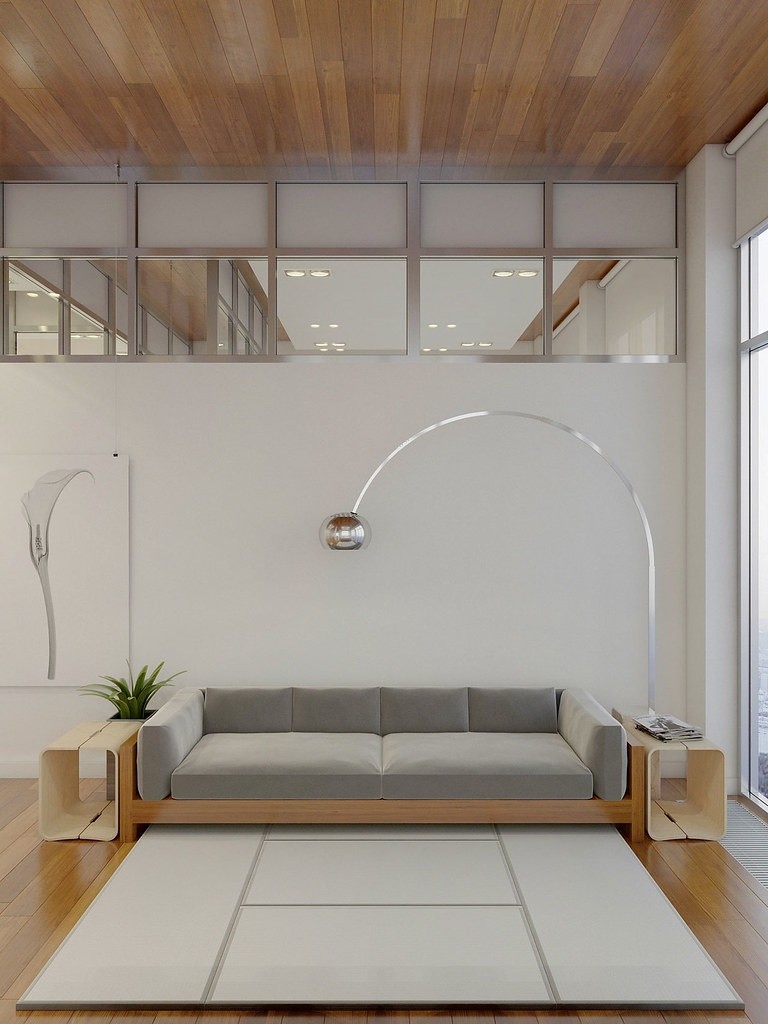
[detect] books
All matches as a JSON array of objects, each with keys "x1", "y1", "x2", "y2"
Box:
[{"x1": 632, "y1": 713, "x2": 703, "y2": 743}]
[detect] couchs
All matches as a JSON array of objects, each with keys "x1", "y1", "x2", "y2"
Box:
[{"x1": 118, "y1": 684, "x2": 647, "y2": 843}]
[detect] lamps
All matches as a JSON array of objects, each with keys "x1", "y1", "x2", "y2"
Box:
[{"x1": 320, "y1": 410, "x2": 657, "y2": 713}]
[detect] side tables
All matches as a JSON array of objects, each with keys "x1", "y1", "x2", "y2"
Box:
[
  {"x1": 623, "y1": 712, "x2": 727, "y2": 839},
  {"x1": 35, "y1": 720, "x2": 141, "y2": 844}
]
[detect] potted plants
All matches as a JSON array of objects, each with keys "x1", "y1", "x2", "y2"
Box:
[{"x1": 78, "y1": 658, "x2": 187, "y2": 801}]
